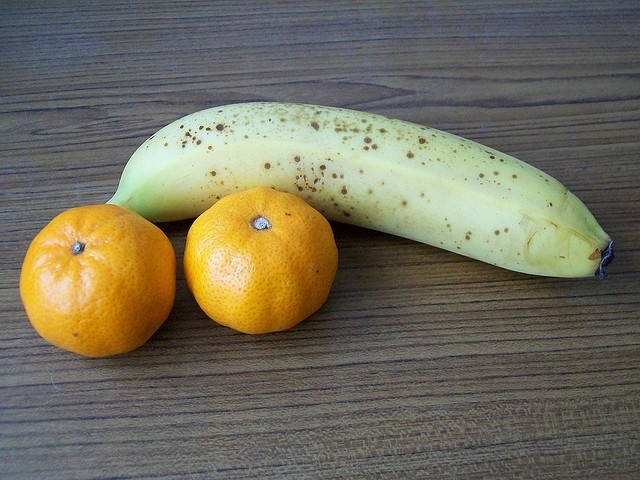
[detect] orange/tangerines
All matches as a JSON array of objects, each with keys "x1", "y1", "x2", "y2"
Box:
[
  {"x1": 183, "y1": 187, "x2": 339, "y2": 334},
  {"x1": 20, "y1": 204, "x2": 176, "y2": 359}
]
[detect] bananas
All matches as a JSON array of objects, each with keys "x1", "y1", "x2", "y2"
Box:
[{"x1": 103, "y1": 101, "x2": 614, "y2": 281}]
